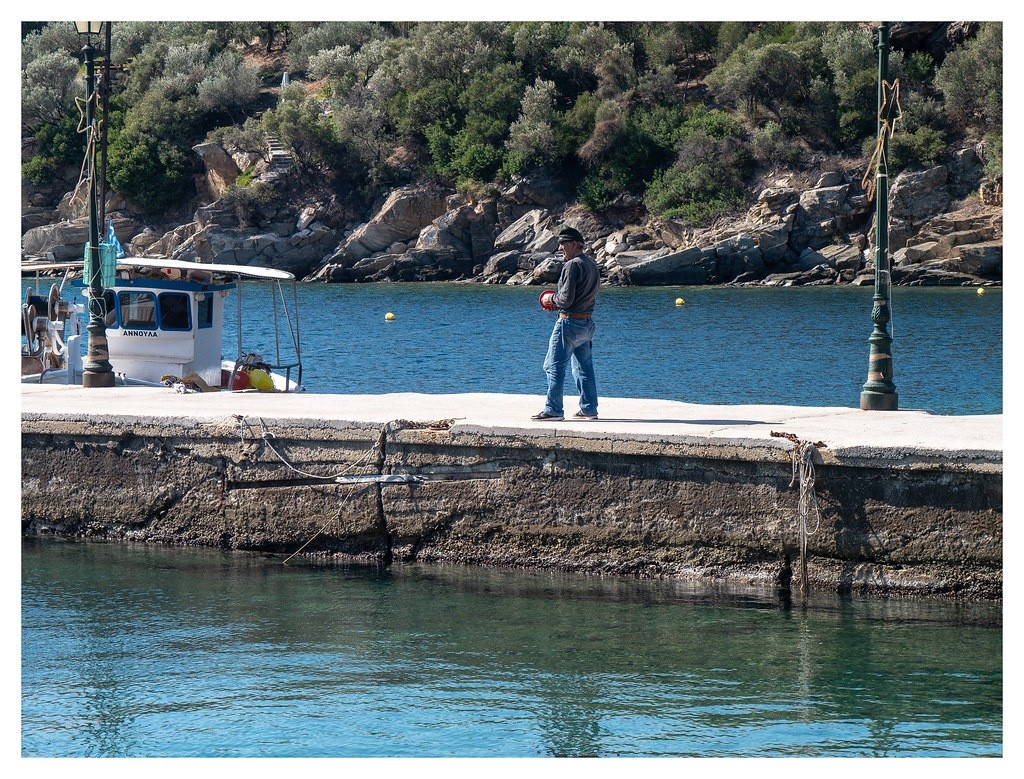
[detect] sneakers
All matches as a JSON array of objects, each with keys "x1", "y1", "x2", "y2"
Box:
[
  {"x1": 531, "y1": 411, "x2": 565, "y2": 421},
  {"x1": 573, "y1": 413, "x2": 598, "y2": 419}
]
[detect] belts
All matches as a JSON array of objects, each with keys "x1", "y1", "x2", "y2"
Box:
[{"x1": 560, "y1": 314, "x2": 591, "y2": 319}]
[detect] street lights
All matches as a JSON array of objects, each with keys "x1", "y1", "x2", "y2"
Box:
[{"x1": 72, "y1": 21, "x2": 115, "y2": 388}]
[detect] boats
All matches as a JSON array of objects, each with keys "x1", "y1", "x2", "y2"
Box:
[{"x1": 22, "y1": 253, "x2": 306, "y2": 395}]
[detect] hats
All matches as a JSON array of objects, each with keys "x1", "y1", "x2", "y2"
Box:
[{"x1": 558, "y1": 228, "x2": 584, "y2": 248}]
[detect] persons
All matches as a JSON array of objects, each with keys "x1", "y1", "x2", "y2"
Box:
[{"x1": 531, "y1": 226, "x2": 600, "y2": 421}]
